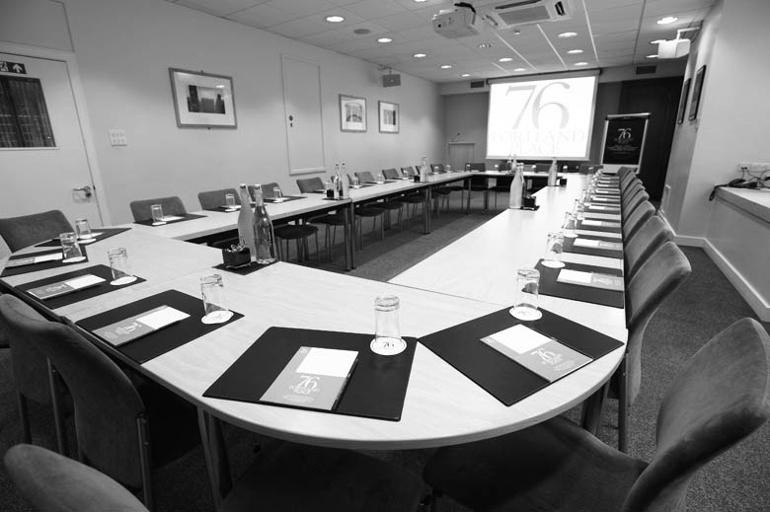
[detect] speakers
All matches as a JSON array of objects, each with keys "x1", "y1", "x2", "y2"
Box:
[
  {"x1": 382, "y1": 65, "x2": 404, "y2": 88},
  {"x1": 657, "y1": 39, "x2": 692, "y2": 60}
]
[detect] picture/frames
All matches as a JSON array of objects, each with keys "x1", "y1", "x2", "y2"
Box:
[
  {"x1": 688, "y1": 64, "x2": 705, "y2": 120},
  {"x1": 168, "y1": 66, "x2": 237, "y2": 128},
  {"x1": 378, "y1": 100, "x2": 399, "y2": 133},
  {"x1": 339, "y1": 94, "x2": 367, "y2": 133},
  {"x1": 675, "y1": 77, "x2": 691, "y2": 124}
]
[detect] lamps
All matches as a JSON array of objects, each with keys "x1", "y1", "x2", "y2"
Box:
[
  {"x1": 377, "y1": 66, "x2": 401, "y2": 88},
  {"x1": 657, "y1": 26, "x2": 700, "y2": 60}
]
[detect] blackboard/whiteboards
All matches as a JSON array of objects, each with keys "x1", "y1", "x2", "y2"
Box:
[{"x1": 601, "y1": 115, "x2": 648, "y2": 167}]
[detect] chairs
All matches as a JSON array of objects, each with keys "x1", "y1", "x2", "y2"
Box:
[
  {"x1": 1, "y1": 209, "x2": 73, "y2": 253},
  {"x1": 494, "y1": 164, "x2": 514, "y2": 206},
  {"x1": 5, "y1": 326, "x2": 54, "y2": 445},
  {"x1": 619, "y1": 241, "x2": 692, "y2": 407},
  {"x1": 331, "y1": 174, "x2": 385, "y2": 248},
  {"x1": 423, "y1": 317, "x2": 770, "y2": 510},
  {"x1": 462, "y1": 162, "x2": 489, "y2": 211},
  {"x1": 416, "y1": 165, "x2": 437, "y2": 216},
  {"x1": 402, "y1": 165, "x2": 424, "y2": 223},
  {"x1": 2, "y1": 442, "x2": 160, "y2": 511},
  {"x1": 619, "y1": 164, "x2": 649, "y2": 210},
  {"x1": 353, "y1": 172, "x2": 403, "y2": 233},
  {"x1": 198, "y1": 188, "x2": 241, "y2": 210},
  {"x1": 1, "y1": 294, "x2": 163, "y2": 509},
  {"x1": 130, "y1": 197, "x2": 186, "y2": 223},
  {"x1": 623, "y1": 200, "x2": 656, "y2": 238},
  {"x1": 296, "y1": 175, "x2": 357, "y2": 265},
  {"x1": 430, "y1": 163, "x2": 451, "y2": 212},
  {"x1": 523, "y1": 162, "x2": 550, "y2": 206},
  {"x1": 382, "y1": 168, "x2": 423, "y2": 221},
  {"x1": 625, "y1": 217, "x2": 678, "y2": 272},
  {"x1": 249, "y1": 181, "x2": 320, "y2": 269}
]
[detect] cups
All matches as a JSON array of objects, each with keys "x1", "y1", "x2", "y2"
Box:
[
  {"x1": 59, "y1": 232, "x2": 83, "y2": 260},
  {"x1": 225, "y1": 194, "x2": 235, "y2": 210},
  {"x1": 445, "y1": 165, "x2": 451, "y2": 173},
  {"x1": 376, "y1": 173, "x2": 384, "y2": 184},
  {"x1": 564, "y1": 211, "x2": 575, "y2": 236},
  {"x1": 74, "y1": 217, "x2": 92, "y2": 242},
  {"x1": 434, "y1": 166, "x2": 439, "y2": 175},
  {"x1": 200, "y1": 274, "x2": 226, "y2": 320},
  {"x1": 351, "y1": 178, "x2": 358, "y2": 189},
  {"x1": 513, "y1": 268, "x2": 539, "y2": 318},
  {"x1": 466, "y1": 164, "x2": 471, "y2": 173},
  {"x1": 581, "y1": 189, "x2": 590, "y2": 207},
  {"x1": 374, "y1": 295, "x2": 401, "y2": 351},
  {"x1": 587, "y1": 168, "x2": 602, "y2": 194},
  {"x1": 151, "y1": 205, "x2": 164, "y2": 222},
  {"x1": 545, "y1": 232, "x2": 563, "y2": 265},
  {"x1": 548, "y1": 158, "x2": 557, "y2": 186},
  {"x1": 274, "y1": 187, "x2": 281, "y2": 202},
  {"x1": 573, "y1": 199, "x2": 585, "y2": 220},
  {"x1": 107, "y1": 249, "x2": 131, "y2": 282}
]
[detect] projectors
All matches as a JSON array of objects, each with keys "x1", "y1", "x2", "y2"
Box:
[{"x1": 429, "y1": 1, "x2": 486, "y2": 41}]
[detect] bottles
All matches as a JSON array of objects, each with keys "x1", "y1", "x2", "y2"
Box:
[
  {"x1": 421, "y1": 158, "x2": 427, "y2": 183},
  {"x1": 252, "y1": 184, "x2": 276, "y2": 264},
  {"x1": 340, "y1": 163, "x2": 349, "y2": 200},
  {"x1": 509, "y1": 163, "x2": 523, "y2": 209},
  {"x1": 520, "y1": 163, "x2": 526, "y2": 209},
  {"x1": 512, "y1": 154, "x2": 517, "y2": 173},
  {"x1": 333, "y1": 164, "x2": 343, "y2": 200},
  {"x1": 238, "y1": 183, "x2": 257, "y2": 263}
]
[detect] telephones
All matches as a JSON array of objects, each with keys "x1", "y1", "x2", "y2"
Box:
[{"x1": 728, "y1": 179, "x2": 757, "y2": 190}]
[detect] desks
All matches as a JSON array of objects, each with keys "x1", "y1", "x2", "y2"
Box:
[
  {"x1": 322, "y1": 179, "x2": 429, "y2": 268},
  {"x1": 0, "y1": 225, "x2": 630, "y2": 512},
  {"x1": 407, "y1": 169, "x2": 472, "y2": 231},
  {"x1": 473, "y1": 168, "x2": 563, "y2": 177},
  {"x1": 389, "y1": 172, "x2": 624, "y2": 328},
  {"x1": 114, "y1": 194, "x2": 350, "y2": 266}
]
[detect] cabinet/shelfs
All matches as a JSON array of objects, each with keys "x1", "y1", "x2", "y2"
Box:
[{"x1": 701, "y1": 186, "x2": 770, "y2": 322}]
